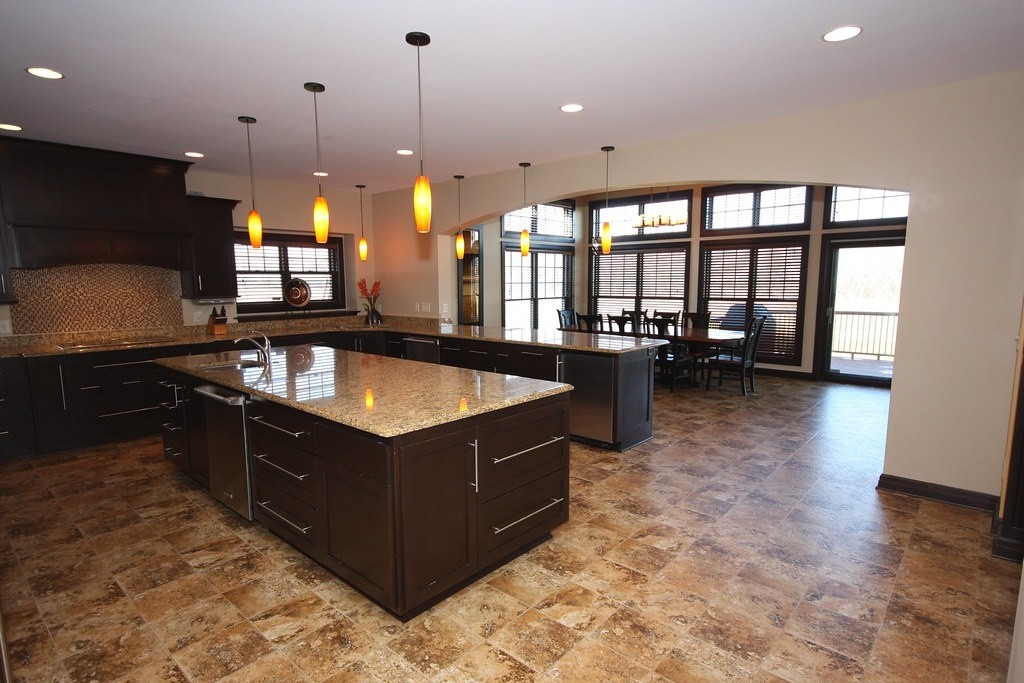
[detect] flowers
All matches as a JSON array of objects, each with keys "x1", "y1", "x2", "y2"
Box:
[{"x1": 357, "y1": 279, "x2": 381, "y2": 298}]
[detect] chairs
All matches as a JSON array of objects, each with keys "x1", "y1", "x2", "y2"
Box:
[{"x1": 557, "y1": 308, "x2": 767, "y2": 396}]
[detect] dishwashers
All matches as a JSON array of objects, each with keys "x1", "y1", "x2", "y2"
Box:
[{"x1": 194, "y1": 384, "x2": 254, "y2": 523}]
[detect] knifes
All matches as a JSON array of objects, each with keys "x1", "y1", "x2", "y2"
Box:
[{"x1": 211, "y1": 305, "x2": 226, "y2": 319}]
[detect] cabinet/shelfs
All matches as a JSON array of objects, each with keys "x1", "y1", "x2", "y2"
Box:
[
  {"x1": 179, "y1": 205, "x2": 237, "y2": 300},
  {"x1": 1, "y1": 330, "x2": 658, "y2": 624}
]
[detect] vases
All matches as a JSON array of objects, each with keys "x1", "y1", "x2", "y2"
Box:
[{"x1": 364, "y1": 296, "x2": 383, "y2": 325}]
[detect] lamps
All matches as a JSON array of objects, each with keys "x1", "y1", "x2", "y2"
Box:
[
  {"x1": 356, "y1": 185, "x2": 368, "y2": 261},
  {"x1": 238, "y1": 116, "x2": 262, "y2": 248},
  {"x1": 519, "y1": 163, "x2": 531, "y2": 256},
  {"x1": 601, "y1": 146, "x2": 615, "y2": 254},
  {"x1": 454, "y1": 175, "x2": 465, "y2": 259},
  {"x1": 304, "y1": 83, "x2": 329, "y2": 243},
  {"x1": 405, "y1": 32, "x2": 431, "y2": 233}
]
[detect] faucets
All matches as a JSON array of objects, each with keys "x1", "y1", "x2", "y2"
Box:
[{"x1": 234, "y1": 331, "x2": 271, "y2": 363}]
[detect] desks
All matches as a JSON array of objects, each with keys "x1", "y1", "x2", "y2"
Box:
[{"x1": 558, "y1": 321, "x2": 755, "y2": 347}]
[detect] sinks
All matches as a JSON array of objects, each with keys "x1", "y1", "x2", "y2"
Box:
[{"x1": 195, "y1": 360, "x2": 271, "y2": 372}]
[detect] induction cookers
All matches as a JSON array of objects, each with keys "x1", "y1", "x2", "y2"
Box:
[{"x1": 53, "y1": 337, "x2": 176, "y2": 351}]
